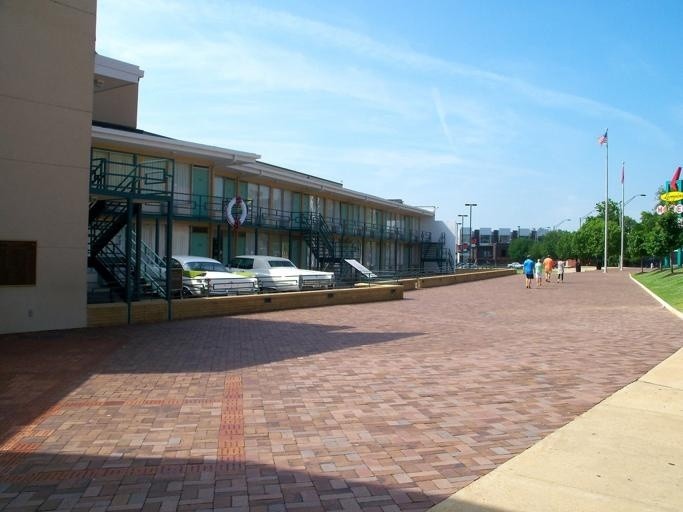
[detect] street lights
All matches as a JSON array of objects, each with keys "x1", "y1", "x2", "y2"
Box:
[{"x1": 619, "y1": 194, "x2": 646, "y2": 227}]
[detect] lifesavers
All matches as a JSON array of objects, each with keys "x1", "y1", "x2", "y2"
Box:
[{"x1": 225, "y1": 197, "x2": 247, "y2": 227}]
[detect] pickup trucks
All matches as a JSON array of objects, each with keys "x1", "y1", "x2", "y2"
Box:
[{"x1": 153, "y1": 255, "x2": 336, "y2": 299}]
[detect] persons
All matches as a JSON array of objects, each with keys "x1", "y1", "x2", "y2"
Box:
[
  {"x1": 523, "y1": 254, "x2": 536, "y2": 289},
  {"x1": 557, "y1": 258, "x2": 564, "y2": 283},
  {"x1": 543, "y1": 253, "x2": 554, "y2": 282},
  {"x1": 535, "y1": 258, "x2": 543, "y2": 288}
]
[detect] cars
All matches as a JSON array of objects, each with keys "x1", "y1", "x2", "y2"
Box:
[
  {"x1": 507, "y1": 262, "x2": 524, "y2": 269},
  {"x1": 459, "y1": 263, "x2": 477, "y2": 268}
]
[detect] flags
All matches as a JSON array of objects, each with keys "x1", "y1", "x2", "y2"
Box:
[
  {"x1": 619, "y1": 166, "x2": 624, "y2": 183},
  {"x1": 599, "y1": 130, "x2": 606, "y2": 145}
]
